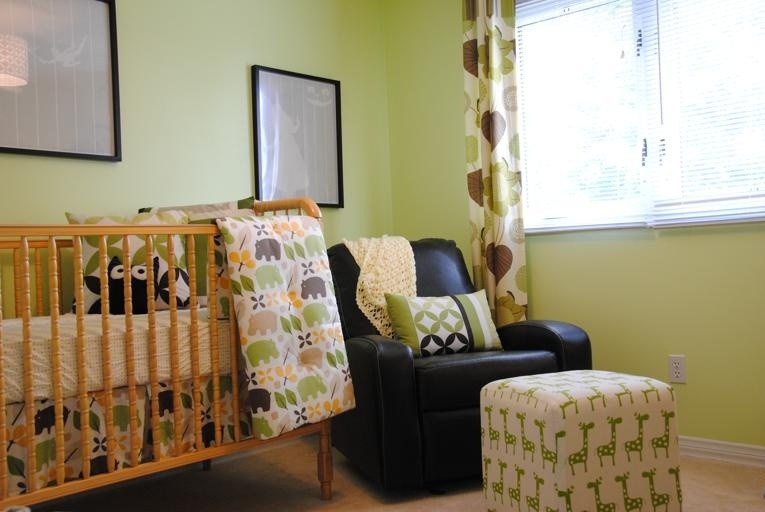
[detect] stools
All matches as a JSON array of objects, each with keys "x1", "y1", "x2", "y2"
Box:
[{"x1": 476, "y1": 368, "x2": 685, "y2": 511}]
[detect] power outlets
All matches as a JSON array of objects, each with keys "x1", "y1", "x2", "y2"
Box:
[{"x1": 666, "y1": 354, "x2": 688, "y2": 386}]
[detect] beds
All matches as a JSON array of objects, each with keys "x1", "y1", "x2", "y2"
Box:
[{"x1": 0, "y1": 195, "x2": 359, "y2": 511}]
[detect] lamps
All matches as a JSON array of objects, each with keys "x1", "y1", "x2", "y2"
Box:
[{"x1": 1, "y1": 33, "x2": 30, "y2": 88}]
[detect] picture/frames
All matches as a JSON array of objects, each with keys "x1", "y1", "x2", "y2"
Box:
[
  {"x1": 250, "y1": 64, "x2": 346, "y2": 209},
  {"x1": 1, "y1": 1, "x2": 123, "y2": 162}
]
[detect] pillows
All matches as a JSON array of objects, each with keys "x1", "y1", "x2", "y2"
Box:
[{"x1": 380, "y1": 287, "x2": 505, "y2": 358}]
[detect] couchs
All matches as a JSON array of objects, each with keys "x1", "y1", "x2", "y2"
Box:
[{"x1": 317, "y1": 235, "x2": 594, "y2": 492}]
